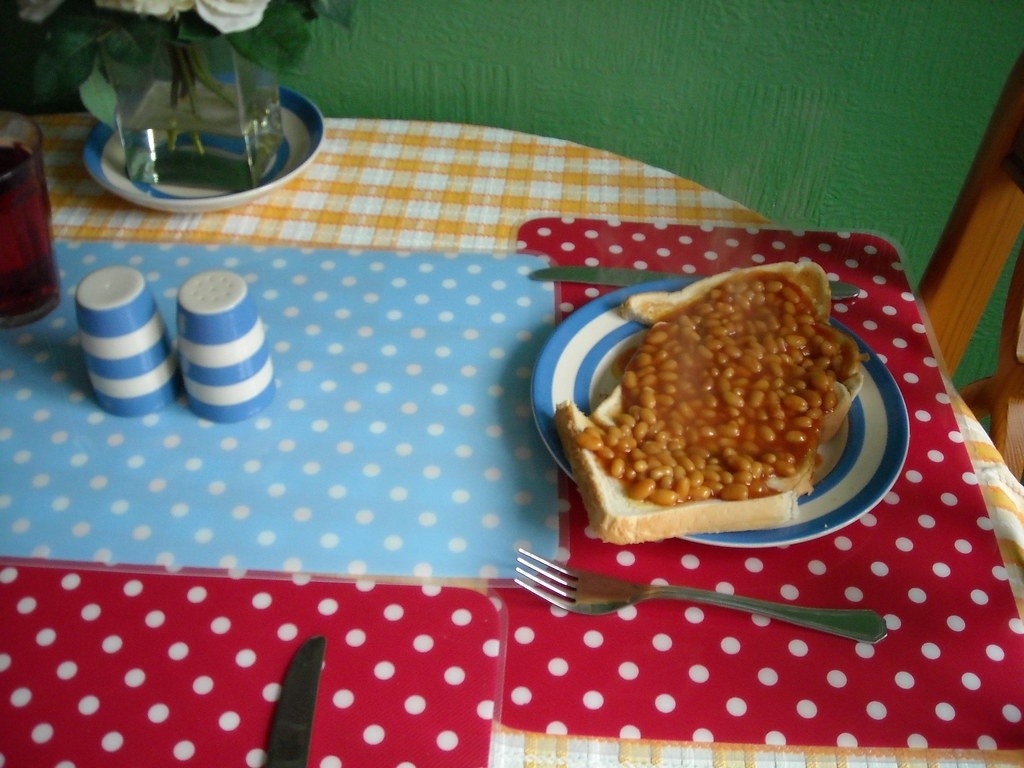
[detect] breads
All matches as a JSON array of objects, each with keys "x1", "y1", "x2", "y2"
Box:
[{"x1": 557, "y1": 258, "x2": 861, "y2": 544}]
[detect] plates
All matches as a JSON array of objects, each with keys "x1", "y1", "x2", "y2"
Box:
[
  {"x1": 529, "y1": 279, "x2": 910, "y2": 548},
  {"x1": 82, "y1": 75, "x2": 323, "y2": 213}
]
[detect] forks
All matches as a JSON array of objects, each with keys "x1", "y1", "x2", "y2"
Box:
[{"x1": 513, "y1": 547, "x2": 887, "y2": 644}]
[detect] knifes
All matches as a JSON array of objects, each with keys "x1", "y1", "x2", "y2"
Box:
[
  {"x1": 265, "y1": 637, "x2": 325, "y2": 768},
  {"x1": 529, "y1": 263, "x2": 859, "y2": 300}
]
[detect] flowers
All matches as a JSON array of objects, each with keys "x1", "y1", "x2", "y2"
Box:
[{"x1": 15, "y1": 0, "x2": 273, "y2": 35}]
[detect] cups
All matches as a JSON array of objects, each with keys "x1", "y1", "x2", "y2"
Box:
[
  {"x1": 177, "y1": 270, "x2": 277, "y2": 424},
  {"x1": 74, "y1": 265, "x2": 177, "y2": 418},
  {"x1": 0, "y1": 111, "x2": 61, "y2": 328}
]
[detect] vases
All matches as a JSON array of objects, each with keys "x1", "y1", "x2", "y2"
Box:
[{"x1": 99, "y1": 30, "x2": 285, "y2": 190}]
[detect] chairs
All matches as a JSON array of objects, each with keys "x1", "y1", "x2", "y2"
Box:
[{"x1": 918, "y1": 51, "x2": 1024, "y2": 486}]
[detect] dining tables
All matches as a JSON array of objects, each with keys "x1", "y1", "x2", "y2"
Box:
[{"x1": 0, "y1": 112, "x2": 1024, "y2": 768}]
